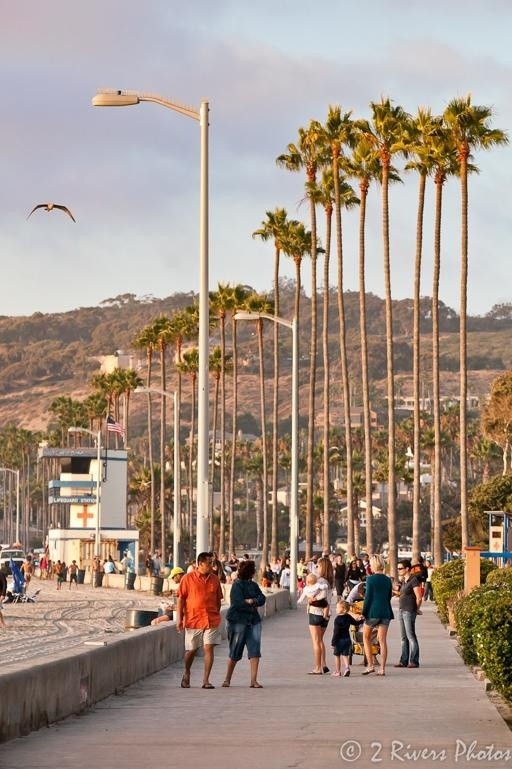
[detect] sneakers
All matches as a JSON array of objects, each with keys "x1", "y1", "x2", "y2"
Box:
[{"x1": 395, "y1": 662, "x2": 419, "y2": 668}]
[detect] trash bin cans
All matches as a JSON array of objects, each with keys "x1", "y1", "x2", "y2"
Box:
[
  {"x1": 124, "y1": 609, "x2": 159, "y2": 633},
  {"x1": 77, "y1": 570, "x2": 85, "y2": 584},
  {"x1": 94, "y1": 571, "x2": 105, "y2": 587},
  {"x1": 150, "y1": 576, "x2": 163, "y2": 596},
  {"x1": 125, "y1": 572, "x2": 136, "y2": 590}
]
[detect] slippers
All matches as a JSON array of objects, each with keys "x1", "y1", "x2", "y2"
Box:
[
  {"x1": 181, "y1": 674, "x2": 190, "y2": 688},
  {"x1": 308, "y1": 666, "x2": 330, "y2": 675},
  {"x1": 202, "y1": 683, "x2": 215, "y2": 689}
]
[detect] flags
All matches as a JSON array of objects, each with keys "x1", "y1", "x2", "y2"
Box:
[{"x1": 107, "y1": 417, "x2": 125, "y2": 437}]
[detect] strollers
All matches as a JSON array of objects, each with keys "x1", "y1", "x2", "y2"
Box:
[{"x1": 343, "y1": 600, "x2": 383, "y2": 667}]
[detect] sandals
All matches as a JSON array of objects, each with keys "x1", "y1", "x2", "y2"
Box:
[
  {"x1": 332, "y1": 671, "x2": 341, "y2": 676},
  {"x1": 344, "y1": 667, "x2": 350, "y2": 676},
  {"x1": 250, "y1": 680, "x2": 263, "y2": 688},
  {"x1": 222, "y1": 680, "x2": 230, "y2": 687},
  {"x1": 376, "y1": 670, "x2": 385, "y2": 675},
  {"x1": 362, "y1": 667, "x2": 375, "y2": 675}
]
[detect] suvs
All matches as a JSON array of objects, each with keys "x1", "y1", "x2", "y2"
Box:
[{"x1": 0, "y1": 549, "x2": 26, "y2": 573}]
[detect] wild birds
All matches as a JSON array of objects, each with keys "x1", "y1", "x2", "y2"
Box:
[{"x1": 27, "y1": 202, "x2": 77, "y2": 222}]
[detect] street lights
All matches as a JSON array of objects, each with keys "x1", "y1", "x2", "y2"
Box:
[
  {"x1": 0, "y1": 467, "x2": 20, "y2": 546},
  {"x1": 67, "y1": 426, "x2": 103, "y2": 564},
  {"x1": 230, "y1": 307, "x2": 303, "y2": 610},
  {"x1": 132, "y1": 383, "x2": 182, "y2": 569},
  {"x1": 90, "y1": 86, "x2": 218, "y2": 575}
]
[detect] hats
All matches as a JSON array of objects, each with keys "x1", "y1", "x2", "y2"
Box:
[{"x1": 168, "y1": 566, "x2": 184, "y2": 579}]
